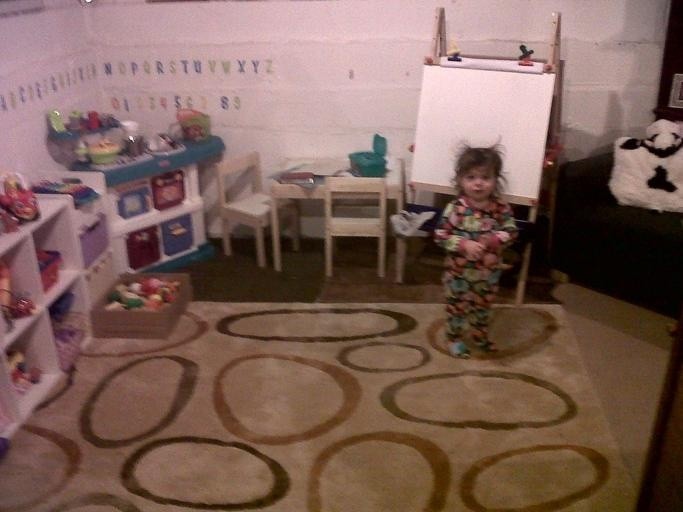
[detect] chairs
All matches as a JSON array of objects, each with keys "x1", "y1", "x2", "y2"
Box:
[
  {"x1": 215, "y1": 150, "x2": 302, "y2": 270},
  {"x1": 321, "y1": 174, "x2": 393, "y2": 283}
]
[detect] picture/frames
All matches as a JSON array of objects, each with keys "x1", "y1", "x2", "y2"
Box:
[{"x1": 667, "y1": 73, "x2": 682, "y2": 109}]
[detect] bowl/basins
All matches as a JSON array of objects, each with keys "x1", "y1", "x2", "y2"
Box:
[{"x1": 89, "y1": 150, "x2": 120, "y2": 165}]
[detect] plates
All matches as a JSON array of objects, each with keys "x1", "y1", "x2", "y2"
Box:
[{"x1": 144, "y1": 143, "x2": 186, "y2": 157}]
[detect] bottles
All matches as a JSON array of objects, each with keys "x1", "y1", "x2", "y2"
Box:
[
  {"x1": 68, "y1": 111, "x2": 80, "y2": 130},
  {"x1": 79, "y1": 113, "x2": 87, "y2": 129}
]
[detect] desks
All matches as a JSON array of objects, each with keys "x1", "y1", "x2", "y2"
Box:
[{"x1": 264, "y1": 153, "x2": 410, "y2": 285}]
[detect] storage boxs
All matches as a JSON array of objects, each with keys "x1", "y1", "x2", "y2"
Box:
[{"x1": 88, "y1": 270, "x2": 194, "y2": 342}]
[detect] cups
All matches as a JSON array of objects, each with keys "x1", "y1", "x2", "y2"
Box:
[
  {"x1": 119, "y1": 120, "x2": 138, "y2": 137},
  {"x1": 122, "y1": 134, "x2": 143, "y2": 156},
  {"x1": 87, "y1": 111, "x2": 99, "y2": 130}
]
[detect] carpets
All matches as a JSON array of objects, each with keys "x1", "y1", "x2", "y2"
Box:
[{"x1": 0, "y1": 287, "x2": 645, "y2": 512}]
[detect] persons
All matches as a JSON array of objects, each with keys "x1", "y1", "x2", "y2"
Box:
[{"x1": 433, "y1": 134, "x2": 519, "y2": 359}]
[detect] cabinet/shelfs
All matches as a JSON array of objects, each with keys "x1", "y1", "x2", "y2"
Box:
[
  {"x1": 21, "y1": 169, "x2": 117, "y2": 318},
  {"x1": 0, "y1": 184, "x2": 99, "y2": 453},
  {"x1": 71, "y1": 129, "x2": 228, "y2": 275}
]
